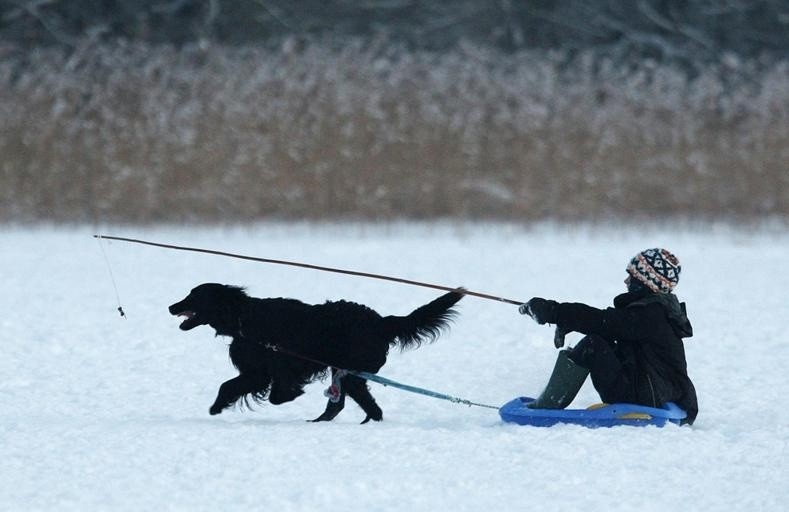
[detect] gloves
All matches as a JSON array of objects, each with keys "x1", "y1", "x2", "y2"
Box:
[{"x1": 517, "y1": 296, "x2": 563, "y2": 326}]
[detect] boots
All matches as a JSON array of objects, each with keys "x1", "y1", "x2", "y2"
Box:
[{"x1": 521, "y1": 350, "x2": 591, "y2": 412}]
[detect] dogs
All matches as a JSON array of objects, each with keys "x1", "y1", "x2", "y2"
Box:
[{"x1": 169, "y1": 283, "x2": 468, "y2": 423}]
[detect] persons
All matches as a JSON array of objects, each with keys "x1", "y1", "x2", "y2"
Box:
[{"x1": 519, "y1": 248, "x2": 697, "y2": 425}]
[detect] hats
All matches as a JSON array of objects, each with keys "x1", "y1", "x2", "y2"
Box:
[{"x1": 625, "y1": 246, "x2": 683, "y2": 298}]
